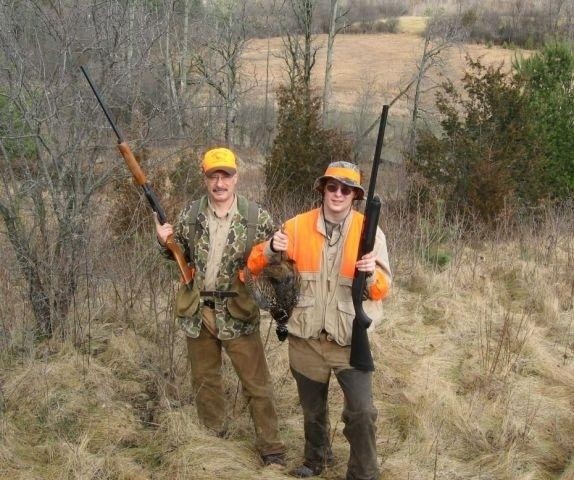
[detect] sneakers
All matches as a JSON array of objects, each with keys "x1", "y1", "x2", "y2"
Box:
[
  {"x1": 262, "y1": 454, "x2": 286, "y2": 471},
  {"x1": 289, "y1": 454, "x2": 334, "y2": 478}
]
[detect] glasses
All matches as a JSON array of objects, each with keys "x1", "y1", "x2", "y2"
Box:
[{"x1": 324, "y1": 184, "x2": 354, "y2": 197}]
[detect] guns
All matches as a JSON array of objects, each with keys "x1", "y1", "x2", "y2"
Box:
[
  {"x1": 348, "y1": 106, "x2": 390, "y2": 370},
  {"x1": 80, "y1": 64, "x2": 194, "y2": 292}
]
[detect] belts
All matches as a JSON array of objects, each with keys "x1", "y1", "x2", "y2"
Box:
[{"x1": 200, "y1": 291, "x2": 238, "y2": 297}]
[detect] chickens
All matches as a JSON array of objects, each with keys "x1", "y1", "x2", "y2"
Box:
[{"x1": 243, "y1": 223, "x2": 302, "y2": 341}]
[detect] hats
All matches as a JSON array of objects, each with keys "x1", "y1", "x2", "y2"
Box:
[
  {"x1": 201, "y1": 147, "x2": 237, "y2": 176},
  {"x1": 312, "y1": 160, "x2": 365, "y2": 201}
]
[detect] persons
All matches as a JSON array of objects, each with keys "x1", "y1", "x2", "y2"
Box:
[
  {"x1": 247, "y1": 161, "x2": 392, "y2": 480},
  {"x1": 152, "y1": 147, "x2": 287, "y2": 469}
]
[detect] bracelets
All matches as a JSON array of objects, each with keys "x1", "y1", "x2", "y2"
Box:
[{"x1": 270, "y1": 238, "x2": 279, "y2": 253}]
